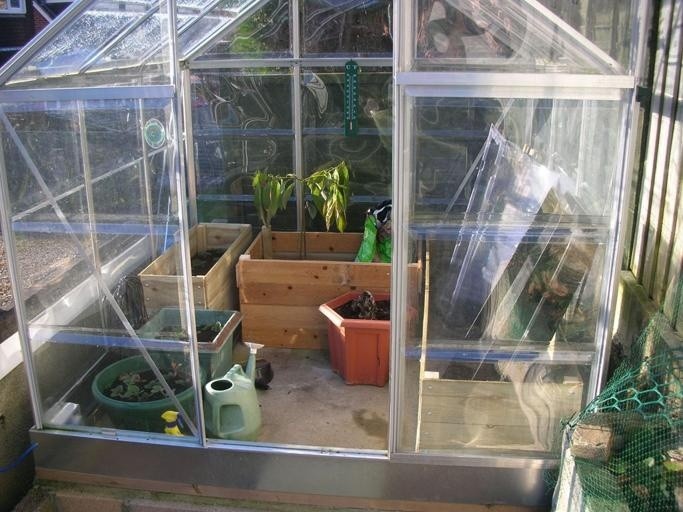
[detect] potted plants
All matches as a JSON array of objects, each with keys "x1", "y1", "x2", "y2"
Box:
[
  {"x1": 231, "y1": 159, "x2": 422, "y2": 351},
  {"x1": 316, "y1": 288, "x2": 417, "y2": 387},
  {"x1": 88, "y1": 304, "x2": 243, "y2": 438}
]
